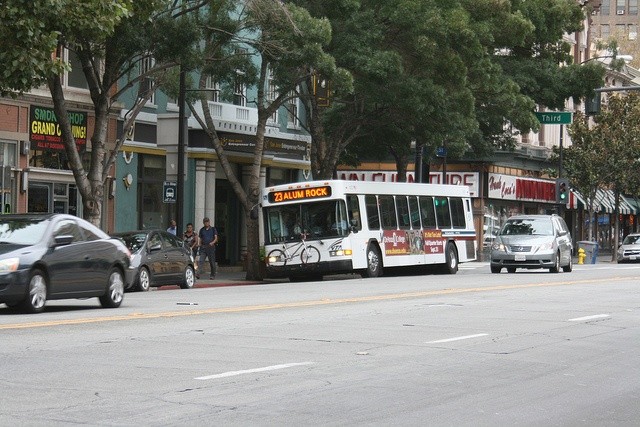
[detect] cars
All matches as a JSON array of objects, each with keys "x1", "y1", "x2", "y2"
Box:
[
  {"x1": 616, "y1": 234, "x2": 640, "y2": 263},
  {"x1": 0, "y1": 213, "x2": 134, "y2": 314},
  {"x1": 108, "y1": 230, "x2": 195, "y2": 292}
]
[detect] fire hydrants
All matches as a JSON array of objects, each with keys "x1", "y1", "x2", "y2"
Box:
[{"x1": 578, "y1": 248, "x2": 586, "y2": 264}]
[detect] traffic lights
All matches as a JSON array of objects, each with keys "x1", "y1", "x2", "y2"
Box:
[{"x1": 555, "y1": 178, "x2": 569, "y2": 204}]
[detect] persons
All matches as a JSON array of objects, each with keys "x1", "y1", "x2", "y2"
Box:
[
  {"x1": 405, "y1": 231, "x2": 425, "y2": 252},
  {"x1": 195, "y1": 217, "x2": 218, "y2": 279},
  {"x1": 183, "y1": 223, "x2": 198, "y2": 261},
  {"x1": 167, "y1": 220, "x2": 177, "y2": 236}
]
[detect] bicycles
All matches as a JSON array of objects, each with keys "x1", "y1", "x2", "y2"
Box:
[{"x1": 267, "y1": 232, "x2": 320, "y2": 271}]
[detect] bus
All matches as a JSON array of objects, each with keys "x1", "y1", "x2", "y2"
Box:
[{"x1": 262, "y1": 180, "x2": 477, "y2": 282}]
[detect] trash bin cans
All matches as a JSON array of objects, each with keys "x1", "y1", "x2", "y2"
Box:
[{"x1": 577, "y1": 239, "x2": 597, "y2": 263}]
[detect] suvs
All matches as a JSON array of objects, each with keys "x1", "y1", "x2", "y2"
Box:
[{"x1": 491, "y1": 213, "x2": 573, "y2": 273}]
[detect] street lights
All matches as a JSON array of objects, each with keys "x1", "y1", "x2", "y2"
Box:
[{"x1": 559, "y1": 54, "x2": 633, "y2": 218}]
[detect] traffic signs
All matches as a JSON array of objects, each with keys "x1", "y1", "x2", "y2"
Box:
[{"x1": 535, "y1": 112, "x2": 573, "y2": 124}]
